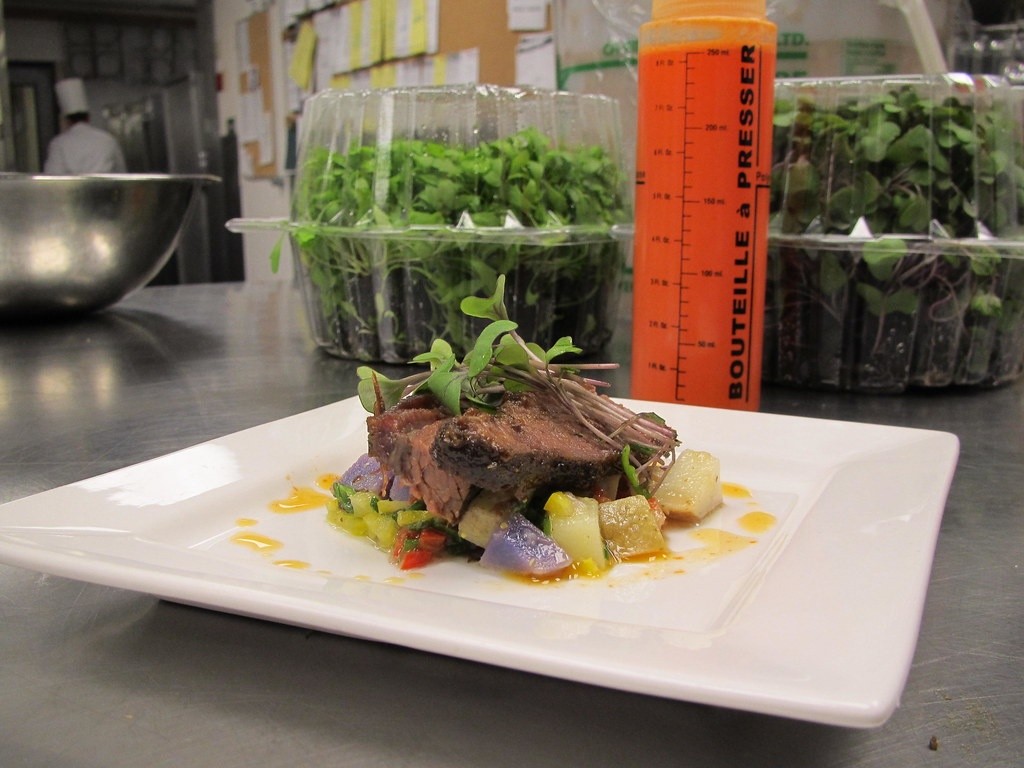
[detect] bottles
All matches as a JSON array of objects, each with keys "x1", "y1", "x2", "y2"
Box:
[{"x1": 631, "y1": 0, "x2": 777, "y2": 413}]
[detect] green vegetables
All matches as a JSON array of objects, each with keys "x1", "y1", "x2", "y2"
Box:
[{"x1": 355, "y1": 274, "x2": 679, "y2": 499}]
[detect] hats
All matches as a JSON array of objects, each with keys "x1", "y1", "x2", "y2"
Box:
[{"x1": 55, "y1": 77, "x2": 89, "y2": 117}]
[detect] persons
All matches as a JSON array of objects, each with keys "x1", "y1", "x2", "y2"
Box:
[{"x1": 43, "y1": 77, "x2": 126, "y2": 175}]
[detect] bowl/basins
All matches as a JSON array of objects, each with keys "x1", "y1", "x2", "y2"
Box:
[{"x1": 0, "y1": 173, "x2": 224, "y2": 316}]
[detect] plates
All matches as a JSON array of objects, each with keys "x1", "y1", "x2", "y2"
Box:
[{"x1": 0, "y1": 395, "x2": 960, "y2": 730}]
